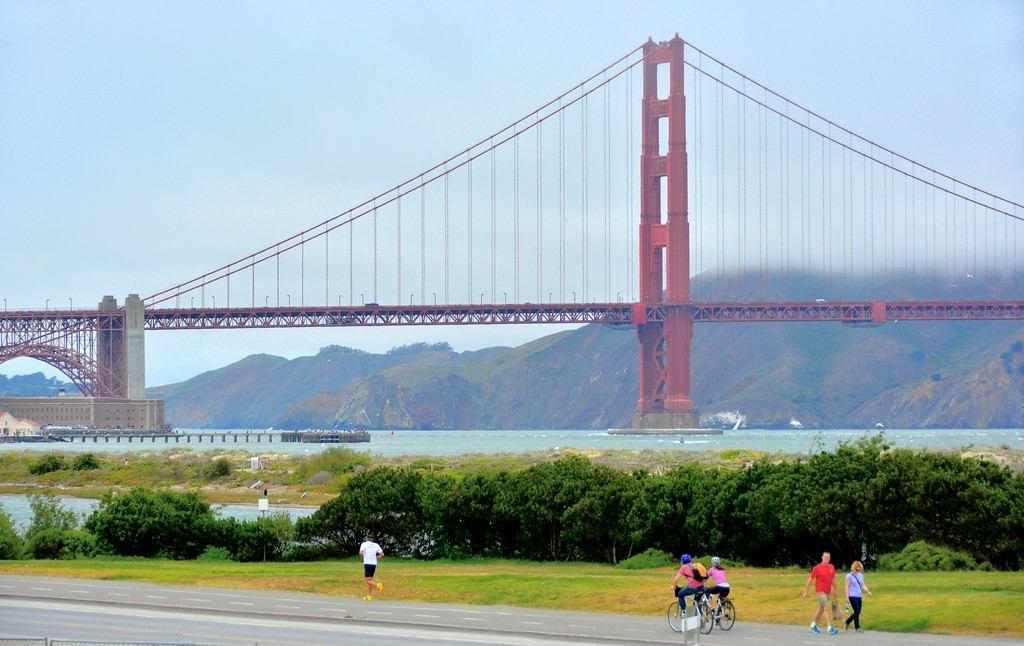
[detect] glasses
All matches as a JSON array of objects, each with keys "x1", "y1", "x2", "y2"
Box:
[{"x1": 856, "y1": 569, "x2": 860, "y2": 572}]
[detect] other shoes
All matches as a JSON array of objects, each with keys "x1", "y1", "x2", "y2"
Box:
[
  {"x1": 363, "y1": 596, "x2": 373, "y2": 600},
  {"x1": 679, "y1": 610, "x2": 686, "y2": 619},
  {"x1": 378, "y1": 583, "x2": 384, "y2": 593}
]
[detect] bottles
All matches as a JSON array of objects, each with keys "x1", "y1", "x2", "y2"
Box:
[{"x1": 846, "y1": 602, "x2": 849, "y2": 612}]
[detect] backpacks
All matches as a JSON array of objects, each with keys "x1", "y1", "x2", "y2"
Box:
[{"x1": 684, "y1": 562, "x2": 708, "y2": 581}]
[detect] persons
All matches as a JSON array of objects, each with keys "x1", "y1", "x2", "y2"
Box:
[
  {"x1": 845, "y1": 561, "x2": 874, "y2": 632},
  {"x1": 670, "y1": 554, "x2": 707, "y2": 618},
  {"x1": 703, "y1": 556, "x2": 730, "y2": 625},
  {"x1": 803, "y1": 552, "x2": 840, "y2": 635},
  {"x1": 359, "y1": 534, "x2": 384, "y2": 601}
]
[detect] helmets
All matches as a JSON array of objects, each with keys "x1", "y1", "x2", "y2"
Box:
[
  {"x1": 711, "y1": 557, "x2": 720, "y2": 565},
  {"x1": 681, "y1": 554, "x2": 691, "y2": 563}
]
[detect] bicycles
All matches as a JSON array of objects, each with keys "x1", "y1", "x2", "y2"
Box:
[
  {"x1": 699, "y1": 585, "x2": 736, "y2": 631},
  {"x1": 665, "y1": 585, "x2": 715, "y2": 635}
]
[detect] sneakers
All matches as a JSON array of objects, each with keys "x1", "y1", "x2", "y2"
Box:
[
  {"x1": 828, "y1": 626, "x2": 838, "y2": 635},
  {"x1": 843, "y1": 619, "x2": 849, "y2": 631},
  {"x1": 855, "y1": 628, "x2": 864, "y2": 634},
  {"x1": 809, "y1": 624, "x2": 820, "y2": 634}
]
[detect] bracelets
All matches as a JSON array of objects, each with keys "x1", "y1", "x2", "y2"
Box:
[{"x1": 866, "y1": 590, "x2": 869, "y2": 593}]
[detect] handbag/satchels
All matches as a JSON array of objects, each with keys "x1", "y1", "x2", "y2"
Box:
[{"x1": 832, "y1": 598, "x2": 844, "y2": 620}]
